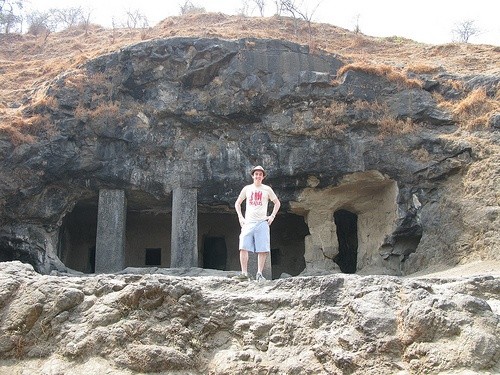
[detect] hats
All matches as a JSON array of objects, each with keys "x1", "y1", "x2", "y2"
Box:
[{"x1": 250, "y1": 165, "x2": 267, "y2": 178}]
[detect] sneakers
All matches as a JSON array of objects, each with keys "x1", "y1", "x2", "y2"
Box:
[
  {"x1": 232, "y1": 273, "x2": 250, "y2": 281},
  {"x1": 256, "y1": 272, "x2": 266, "y2": 281}
]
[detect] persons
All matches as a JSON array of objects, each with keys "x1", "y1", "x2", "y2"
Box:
[{"x1": 235, "y1": 165, "x2": 280, "y2": 280}]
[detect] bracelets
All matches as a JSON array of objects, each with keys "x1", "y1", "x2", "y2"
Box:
[{"x1": 271, "y1": 214, "x2": 275, "y2": 218}]
